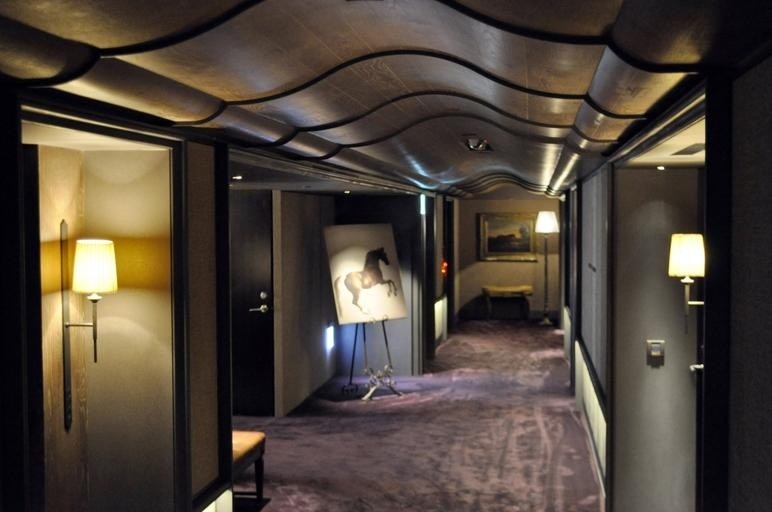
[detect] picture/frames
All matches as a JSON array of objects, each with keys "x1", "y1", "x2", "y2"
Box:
[{"x1": 479, "y1": 211, "x2": 537, "y2": 262}]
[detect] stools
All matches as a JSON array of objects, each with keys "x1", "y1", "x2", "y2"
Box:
[
  {"x1": 481, "y1": 284, "x2": 533, "y2": 319},
  {"x1": 230, "y1": 429, "x2": 267, "y2": 506}
]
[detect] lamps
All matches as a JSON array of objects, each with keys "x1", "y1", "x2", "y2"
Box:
[
  {"x1": 667, "y1": 232, "x2": 706, "y2": 337},
  {"x1": 57, "y1": 219, "x2": 119, "y2": 431},
  {"x1": 534, "y1": 211, "x2": 560, "y2": 324}
]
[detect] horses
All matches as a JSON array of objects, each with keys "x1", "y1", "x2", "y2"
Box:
[{"x1": 333, "y1": 246, "x2": 398, "y2": 319}]
